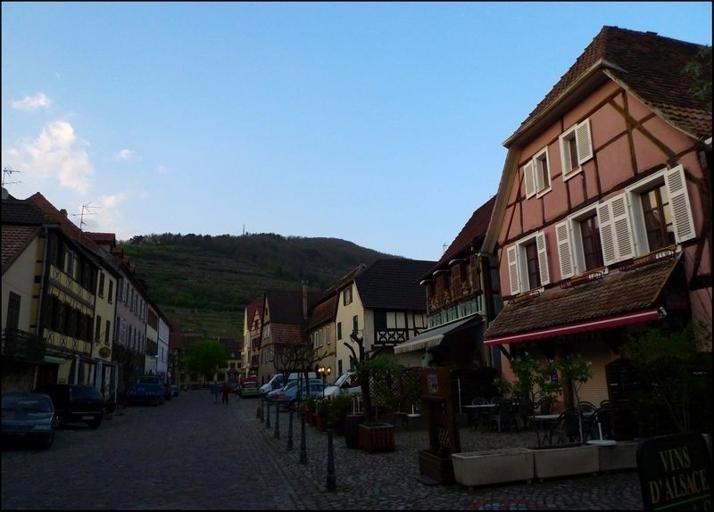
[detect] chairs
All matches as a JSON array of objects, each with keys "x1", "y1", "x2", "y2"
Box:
[{"x1": 473, "y1": 396, "x2": 616, "y2": 446}]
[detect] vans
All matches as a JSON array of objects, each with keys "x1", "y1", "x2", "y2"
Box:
[
  {"x1": 316, "y1": 369, "x2": 362, "y2": 409},
  {"x1": 260, "y1": 371, "x2": 315, "y2": 394}
]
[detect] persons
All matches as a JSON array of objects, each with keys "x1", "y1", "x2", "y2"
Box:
[
  {"x1": 219, "y1": 381, "x2": 229, "y2": 405},
  {"x1": 210, "y1": 380, "x2": 221, "y2": 404}
]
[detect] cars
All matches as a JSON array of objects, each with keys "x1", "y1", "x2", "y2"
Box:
[
  {"x1": 126, "y1": 375, "x2": 177, "y2": 407},
  {"x1": 268, "y1": 379, "x2": 329, "y2": 403},
  {"x1": 239, "y1": 382, "x2": 261, "y2": 397},
  {"x1": 0, "y1": 390, "x2": 59, "y2": 449}
]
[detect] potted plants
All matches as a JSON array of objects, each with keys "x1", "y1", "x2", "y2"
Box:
[{"x1": 296, "y1": 369, "x2": 397, "y2": 452}]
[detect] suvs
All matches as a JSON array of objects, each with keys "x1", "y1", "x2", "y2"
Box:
[{"x1": 44, "y1": 383, "x2": 102, "y2": 430}]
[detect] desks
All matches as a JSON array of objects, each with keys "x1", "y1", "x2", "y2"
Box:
[{"x1": 461, "y1": 404, "x2": 496, "y2": 432}]
